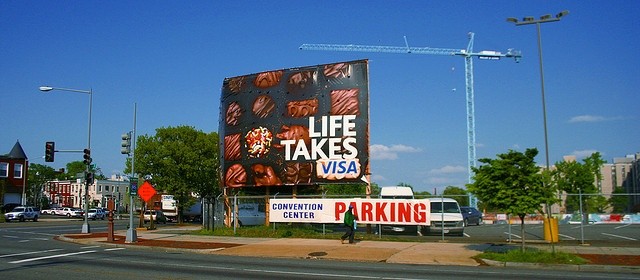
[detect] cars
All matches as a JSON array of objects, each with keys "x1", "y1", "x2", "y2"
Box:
[
  {"x1": 59, "y1": 208, "x2": 81, "y2": 217},
  {"x1": 41, "y1": 208, "x2": 57, "y2": 215},
  {"x1": 51, "y1": 208, "x2": 64, "y2": 215},
  {"x1": 460, "y1": 207, "x2": 483, "y2": 227},
  {"x1": 144, "y1": 210, "x2": 166, "y2": 224}
]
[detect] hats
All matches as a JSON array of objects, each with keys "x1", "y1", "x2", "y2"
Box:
[{"x1": 349, "y1": 206, "x2": 353, "y2": 208}]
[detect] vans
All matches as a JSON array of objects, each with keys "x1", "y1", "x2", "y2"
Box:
[
  {"x1": 380, "y1": 186, "x2": 415, "y2": 232},
  {"x1": 422, "y1": 197, "x2": 465, "y2": 237}
]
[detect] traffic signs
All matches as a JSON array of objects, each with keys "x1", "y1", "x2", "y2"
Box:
[{"x1": 130, "y1": 178, "x2": 138, "y2": 196}]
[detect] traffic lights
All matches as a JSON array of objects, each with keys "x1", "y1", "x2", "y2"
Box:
[
  {"x1": 121, "y1": 131, "x2": 131, "y2": 154},
  {"x1": 83, "y1": 149, "x2": 92, "y2": 165},
  {"x1": 46, "y1": 142, "x2": 54, "y2": 161}
]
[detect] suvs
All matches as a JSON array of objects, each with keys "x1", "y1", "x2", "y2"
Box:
[
  {"x1": 4, "y1": 207, "x2": 40, "y2": 222},
  {"x1": 83, "y1": 209, "x2": 105, "y2": 220}
]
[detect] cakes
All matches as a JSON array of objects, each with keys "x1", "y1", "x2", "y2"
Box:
[
  {"x1": 225, "y1": 78, "x2": 244, "y2": 95},
  {"x1": 251, "y1": 165, "x2": 283, "y2": 186},
  {"x1": 284, "y1": 162, "x2": 313, "y2": 183},
  {"x1": 245, "y1": 126, "x2": 273, "y2": 158},
  {"x1": 287, "y1": 99, "x2": 319, "y2": 117},
  {"x1": 226, "y1": 101, "x2": 241, "y2": 127},
  {"x1": 224, "y1": 133, "x2": 243, "y2": 160},
  {"x1": 252, "y1": 94, "x2": 275, "y2": 119},
  {"x1": 288, "y1": 69, "x2": 313, "y2": 89},
  {"x1": 274, "y1": 124, "x2": 311, "y2": 152},
  {"x1": 324, "y1": 64, "x2": 349, "y2": 80},
  {"x1": 330, "y1": 87, "x2": 360, "y2": 115},
  {"x1": 254, "y1": 70, "x2": 284, "y2": 88},
  {"x1": 226, "y1": 164, "x2": 247, "y2": 187}
]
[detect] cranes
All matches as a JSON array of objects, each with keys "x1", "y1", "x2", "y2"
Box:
[{"x1": 299, "y1": 31, "x2": 523, "y2": 207}]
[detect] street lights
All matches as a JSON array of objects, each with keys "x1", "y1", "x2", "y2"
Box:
[
  {"x1": 39, "y1": 86, "x2": 92, "y2": 233},
  {"x1": 507, "y1": 9, "x2": 569, "y2": 243}
]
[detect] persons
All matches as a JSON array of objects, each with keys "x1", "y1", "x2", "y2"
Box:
[{"x1": 340, "y1": 205, "x2": 359, "y2": 244}]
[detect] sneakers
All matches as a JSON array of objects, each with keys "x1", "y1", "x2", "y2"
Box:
[
  {"x1": 340, "y1": 238, "x2": 344, "y2": 243},
  {"x1": 349, "y1": 241, "x2": 356, "y2": 243}
]
